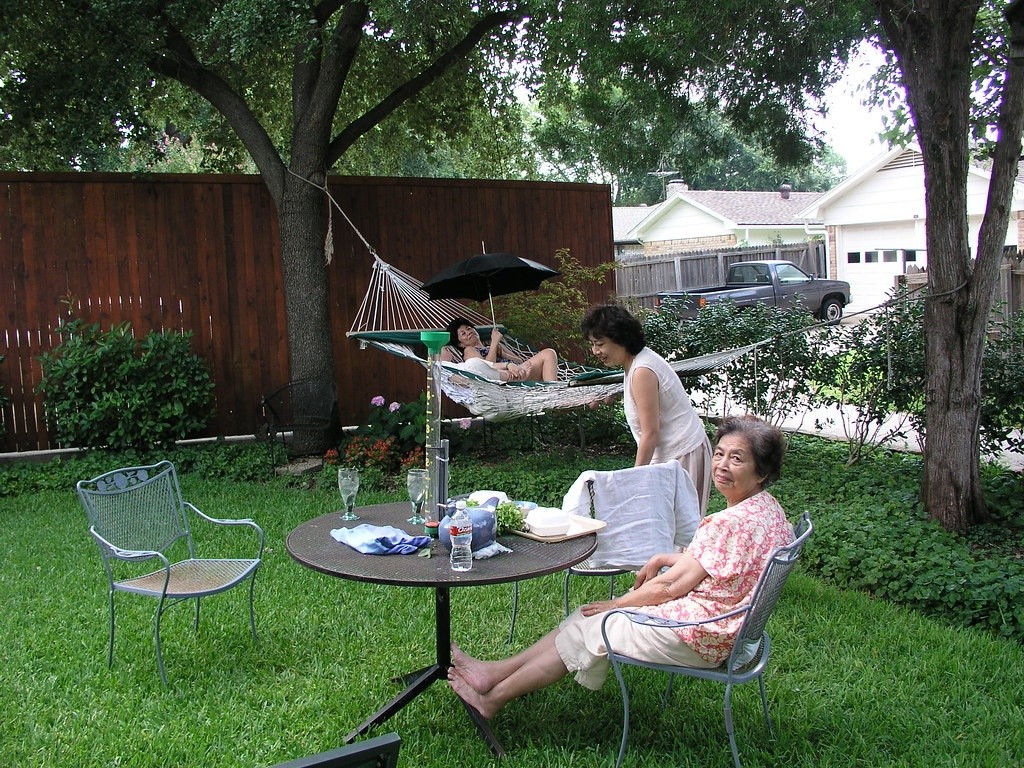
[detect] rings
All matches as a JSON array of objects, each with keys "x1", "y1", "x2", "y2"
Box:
[{"x1": 595, "y1": 608, "x2": 597, "y2": 614}]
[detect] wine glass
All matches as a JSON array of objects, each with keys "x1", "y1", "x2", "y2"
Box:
[
  {"x1": 405, "y1": 469, "x2": 429, "y2": 524},
  {"x1": 338, "y1": 468, "x2": 361, "y2": 520}
]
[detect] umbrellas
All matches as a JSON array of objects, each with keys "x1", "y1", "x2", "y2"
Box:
[{"x1": 419, "y1": 241, "x2": 562, "y2": 359}]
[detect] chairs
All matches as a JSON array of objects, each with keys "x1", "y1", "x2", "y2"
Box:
[
  {"x1": 76, "y1": 459, "x2": 265, "y2": 689},
  {"x1": 508, "y1": 459, "x2": 702, "y2": 644},
  {"x1": 601, "y1": 511, "x2": 813, "y2": 768}
]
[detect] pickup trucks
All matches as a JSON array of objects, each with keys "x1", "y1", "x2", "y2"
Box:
[{"x1": 657, "y1": 259, "x2": 853, "y2": 327}]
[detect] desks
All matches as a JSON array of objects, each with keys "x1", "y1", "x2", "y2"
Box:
[{"x1": 284, "y1": 501, "x2": 599, "y2": 768}]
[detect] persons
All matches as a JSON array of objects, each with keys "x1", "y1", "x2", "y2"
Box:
[
  {"x1": 414, "y1": 317, "x2": 615, "y2": 423},
  {"x1": 582, "y1": 304, "x2": 713, "y2": 592},
  {"x1": 447, "y1": 415, "x2": 798, "y2": 720}
]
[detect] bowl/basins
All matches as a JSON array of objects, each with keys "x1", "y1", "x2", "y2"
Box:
[{"x1": 511, "y1": 500, "x2": 538, "y2": 519}]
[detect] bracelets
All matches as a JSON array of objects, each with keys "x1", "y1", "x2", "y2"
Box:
[{"x1": 505, "y1": 362, "x2": 512, "y2": 370}]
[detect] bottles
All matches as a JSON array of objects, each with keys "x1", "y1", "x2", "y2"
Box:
[{"x1": 450, "y1": 501, "x2": 473, "y2": 572}]
